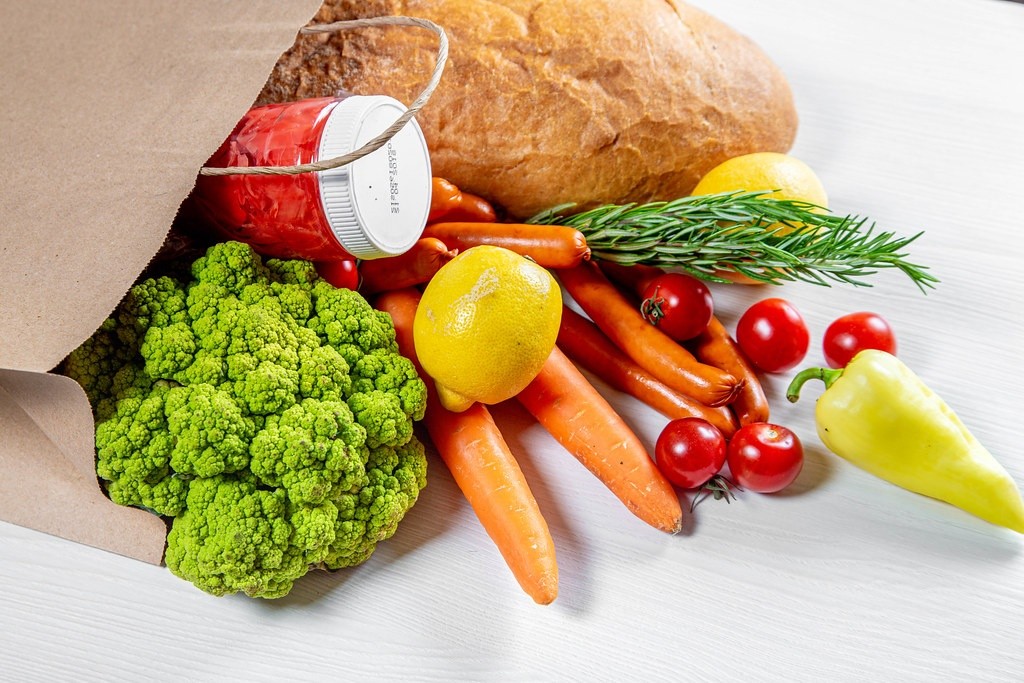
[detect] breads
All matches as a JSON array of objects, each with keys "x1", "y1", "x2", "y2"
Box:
[{"x1": 249, "y1": 0, "x2": 802, "y2": 219}]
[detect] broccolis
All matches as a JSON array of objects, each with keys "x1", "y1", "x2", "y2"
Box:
[{"x1": 57, "y1": 241, "x2": 428, "y2": 600}]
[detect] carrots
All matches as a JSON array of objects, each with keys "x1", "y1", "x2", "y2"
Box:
[{"x1": 374, "y1": 289, "x2": 683, "y2": 605}]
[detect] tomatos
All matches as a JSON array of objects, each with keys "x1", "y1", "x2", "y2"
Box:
[{"x1": 638, "y1": 274, "x2": 900, "y2": 513}]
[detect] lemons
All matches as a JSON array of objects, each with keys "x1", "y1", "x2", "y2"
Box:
[{"x1": 411, "y1": 151, "x2": 830, "y2": 414}]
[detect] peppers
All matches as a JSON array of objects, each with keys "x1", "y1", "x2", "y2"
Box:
[{"x1": 784, "y1": 348, "x2": 1024, "y2": 535}]
